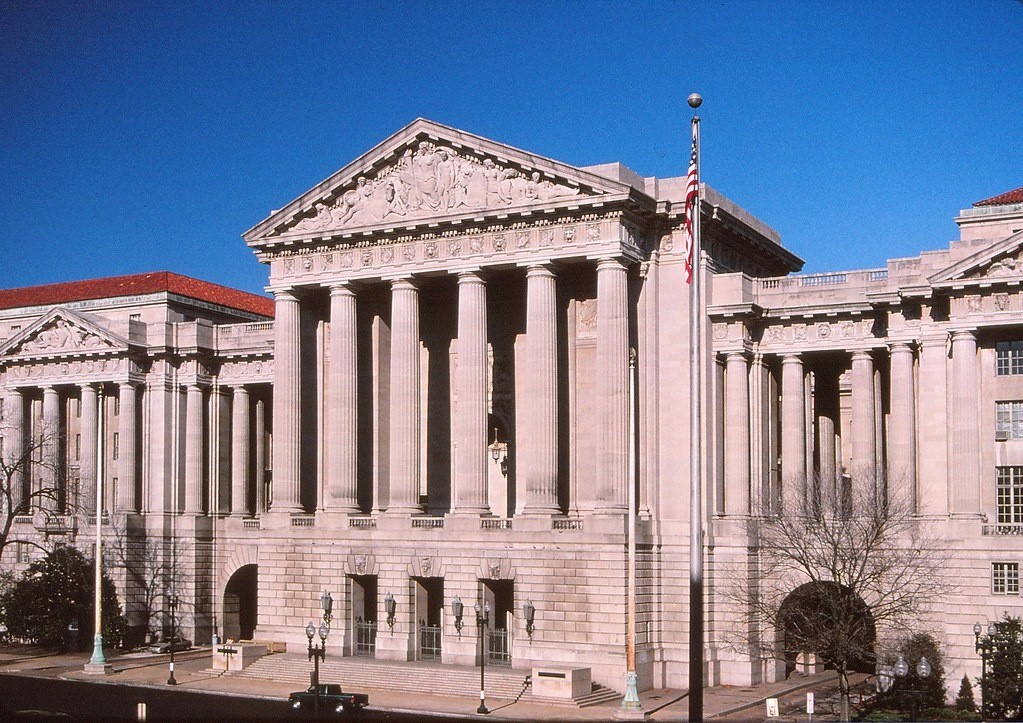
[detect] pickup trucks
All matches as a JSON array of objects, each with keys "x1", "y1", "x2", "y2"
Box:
[{"x1": 287, "y1": 684, "x2": 370, "y2": 713}]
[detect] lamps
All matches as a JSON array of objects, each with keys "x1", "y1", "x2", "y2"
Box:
[
  {"x1": 491, "y1": 428, "x2": 501, "y2": 465},
  {"x1": 385, "y1": 592, "x2": 396, "y2": 636},
  {"x1": 321, "y1": 589, "x2": 333, "y2": 629},
  {"x1": 452, "y1": 595, "x2": 464, "y2": 639},
  {"x1": 522, "y1": 598, "x2": 535, "y2": 644}
]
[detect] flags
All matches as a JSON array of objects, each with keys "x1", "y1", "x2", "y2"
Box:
[{"x1": 685, "y1": 135, "x2": 700, "y2": 284}]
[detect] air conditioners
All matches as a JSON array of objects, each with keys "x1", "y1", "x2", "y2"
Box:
[{"x1": 995, "y1": 430, "x2": 1008, "y2": 440}]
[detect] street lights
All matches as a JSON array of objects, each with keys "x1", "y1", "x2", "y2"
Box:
[
  {"x1": 474, "y1": 604, "x2": 491, "y2": 714},
  {"x1": 306, "y1": 621, "x2": 329, "y2": 723},
  {"x1": 894, "y1": 621, "x2": 997, "y2": 723},
  {"x1": 167, "y1": 589, "x2": 180, "y2": 685}
]
